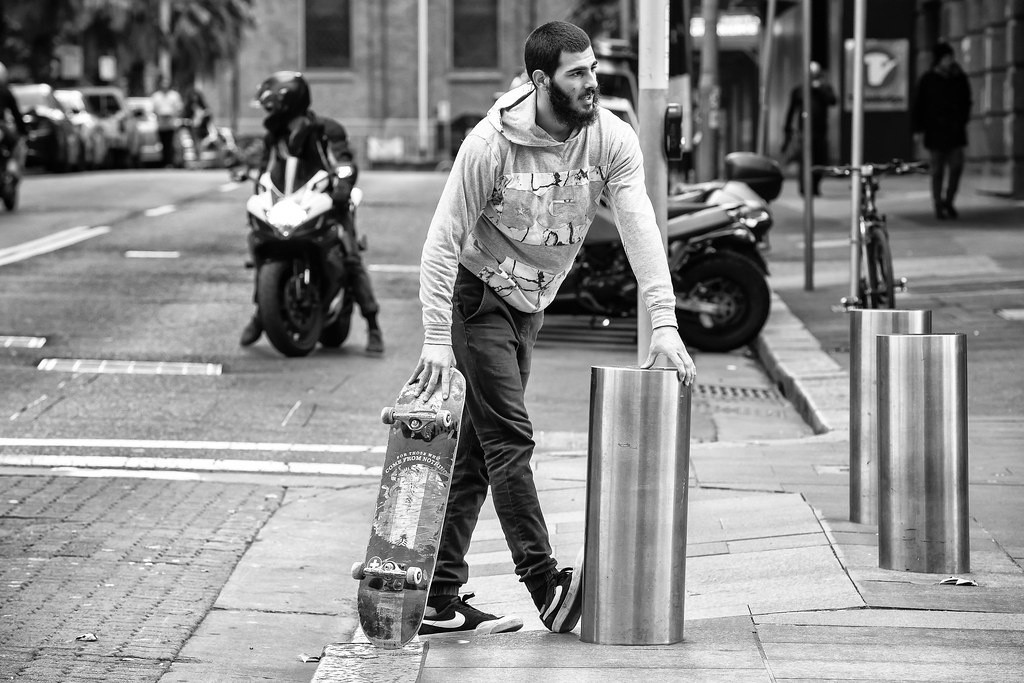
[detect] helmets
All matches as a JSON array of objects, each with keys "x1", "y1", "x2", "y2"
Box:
[{"x1": 254, "y1": 71, "x2": 311, "y2": 115}]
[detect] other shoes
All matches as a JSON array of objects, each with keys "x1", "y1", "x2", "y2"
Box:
[{"x1": 936, "y1": 205, "x2": 959, "y2": 222}]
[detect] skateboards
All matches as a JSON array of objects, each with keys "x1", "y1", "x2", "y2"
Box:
[{"x1": 350, "y1": 361, "x2": 468, "y2": 650}]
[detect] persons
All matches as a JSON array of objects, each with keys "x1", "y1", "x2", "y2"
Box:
[
  {"x1": 180, "y1": 86, "x2": 237, "y2": 169},
  {"x1": 402, "y1": 22, "x2": 697, "y2": 639},
  {"x1": 237, "y1": 70, "x2": 385, "y2": 358},
  {"x1": 912, "y1": 40, "x2": 974, "y2": 220},
  {"x1": 147, "y1": 74, "x2": 185, "y2": 167},
  {"x1": 0, "y1": 60, "x2": 31, "y2": 194},
  {"x1": 779, "y1": 60, "x2": 836, "y2": 198}
]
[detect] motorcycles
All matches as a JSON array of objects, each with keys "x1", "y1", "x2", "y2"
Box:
[
  {"x1": 544, "y1": 151, "x2": 782, "y2": 353},
  {"x1": 0, "y1": 115, "x2": 34, "y2": 210},
  {"x1": 235, "y1": 166, "x2": 369, "y2": 359}
]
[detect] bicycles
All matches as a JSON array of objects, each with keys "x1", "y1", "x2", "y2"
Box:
[{"x1": 814, "y1": 160, "x2": 929, "y2": 309}]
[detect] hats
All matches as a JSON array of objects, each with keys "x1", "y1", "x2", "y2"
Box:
[{"x1": 932, "y1": 40, "x2": 954, "y2": 65}]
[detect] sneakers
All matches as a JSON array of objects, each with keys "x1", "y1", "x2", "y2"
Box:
[
  {"x1": 238, "y1": 313, "x2": 263, "y2": 347},
  {"x1": 539, "y1": 545, "x2": 586, "y2": 634},
  {"x1": 417, "y1": 593, "x2": 523, "y2": 641},
  {"x1": 365, "y1": 327, "x2": 384, "y2": 355}
]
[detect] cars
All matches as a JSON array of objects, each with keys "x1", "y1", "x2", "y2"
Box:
[
  {"x1": 0, "y1": 74, "x2": 239, "y2": 171},
  {"x1": 491, "y1": 61, "x2": 639, "y2": 141}
]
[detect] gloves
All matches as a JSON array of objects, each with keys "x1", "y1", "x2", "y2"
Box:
[{"x1": 331, "y1": 181, "x2": 351, "y2": 218}]
